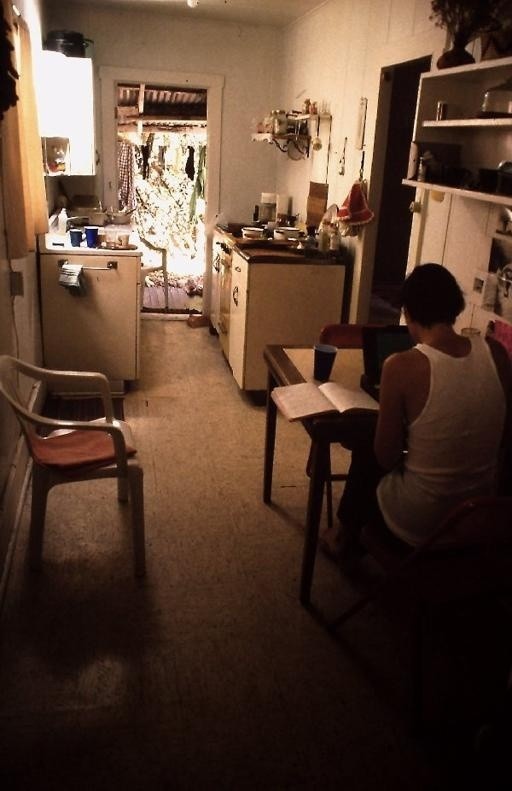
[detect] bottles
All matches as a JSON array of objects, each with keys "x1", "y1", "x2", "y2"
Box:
[
  {"x1": 478, "y1": 77, "x2": 512, "y2": 117},
  {"x1": 260, "y1": 193, "x2": 278, "y2": 230},
  {"x1": 304, "y1": 221, "x2": 340, "y2": 251},
  {"x1": 256, "y1": 98, "x2": 317, "y2": 135},
  {"x1": 435, "y1": 100, "x2": 447, "y2": 121}
]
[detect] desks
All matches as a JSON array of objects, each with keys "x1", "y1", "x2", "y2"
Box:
[{"x1": 261, "y1": 345, "x2": 392, "y2": 601}]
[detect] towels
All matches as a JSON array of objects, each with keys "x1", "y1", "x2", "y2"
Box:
[{"x1": 58, "y1": 264, "x2": 82, "y2": 287}]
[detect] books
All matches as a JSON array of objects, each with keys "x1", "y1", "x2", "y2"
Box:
[{"x1": 269, "y1": 378, "x2": 379, "y2": 423}]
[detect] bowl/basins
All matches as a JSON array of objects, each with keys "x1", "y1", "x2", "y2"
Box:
[
  {"x1": 277, "y1": 215, "x2": 296, "y2": 227},
  {"x1": 272, "y1": 227, "x2": 300, "y2": 240},
  {"x1": 478, "y1": 166, "x2": 511, "y2": 195},
  {"x1": 107, "y1": 214, "x2": 130, "y2": 225},
  {"x1": 417, "y1": 158, "x2": 464, "y2": 187}
]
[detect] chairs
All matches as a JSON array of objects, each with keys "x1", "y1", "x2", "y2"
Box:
[
  {"x1": 358, "y1": 496, "x2": 512, "y2": 637},
  {"x1": 0, "y1": 355, "x2": 146, "y2": 579},
  {"x1": 306, "y1": 325, "x2": 408, "y2": 527}
]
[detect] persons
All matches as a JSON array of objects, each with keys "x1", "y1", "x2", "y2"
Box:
[{"x1": 319, "y1": 264, "x2": 510, "y2": 624}]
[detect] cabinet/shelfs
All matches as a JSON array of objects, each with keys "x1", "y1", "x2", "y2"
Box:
[
  {"x1": 207, "y1": 223, "x2": 346, "y2": 391},
  {"x1": 402, "y1": 57, "x2": 512, "y2": 208},
  {"x1": 249, "y1": 109, "x2": 331, "y2": 157},
  {"x1": 36, "y1": 50, "x2": 101, "y2": 177},
  {"x1": 472, "y1": 232, "x2": 512, "y2": 337},
  {"x1": 38, "y1": 255, "x2": 140, "y2": 397}
]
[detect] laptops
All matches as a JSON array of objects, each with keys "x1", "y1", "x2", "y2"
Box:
[{"x1": 360, "y1": 326, "x2": 416, "y2": 403}]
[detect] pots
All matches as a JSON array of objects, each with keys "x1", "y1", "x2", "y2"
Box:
[{"x1": 66, "y1": 212, "x2": 106, "y2": 227}]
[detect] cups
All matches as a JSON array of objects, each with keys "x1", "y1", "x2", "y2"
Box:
[
  {"x1": 84, "y1": 226, "x2": 100, "y2": 248},
  {"x1": 312, "y1": 343, "x2": 338, "y2": 383},
  {"x1": 121, "y1": 235, "x2": 130, "y2": 246},
  {"x1": 70, "y1": 230, "x2": 85, "y2": 246},
  {"x1": 241, "y1": 227, "x2": 264, "y2": 240}
]
